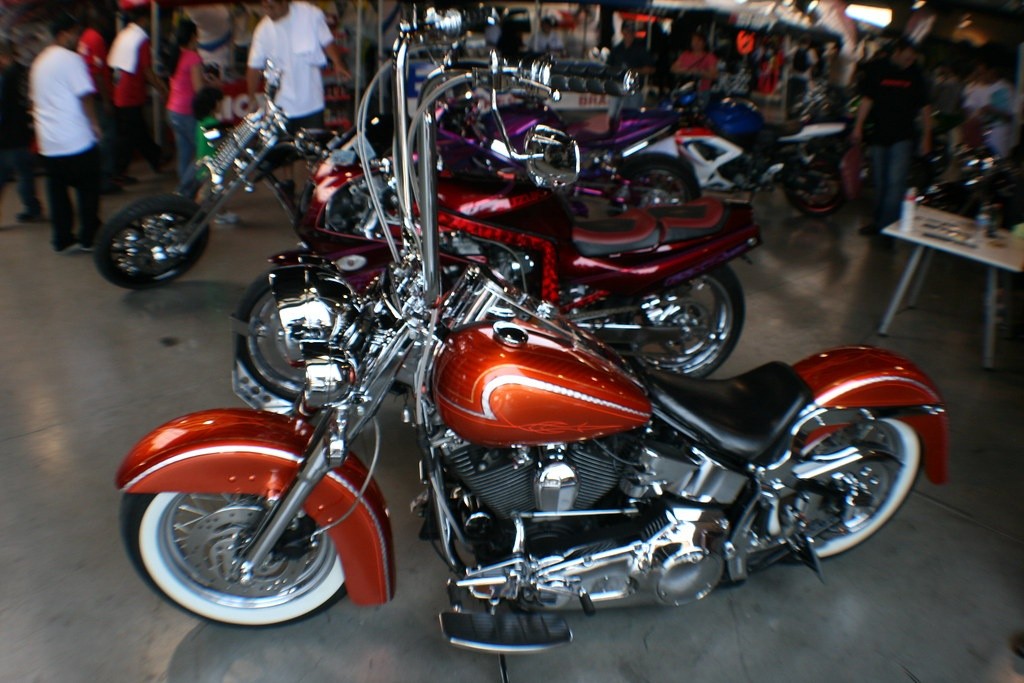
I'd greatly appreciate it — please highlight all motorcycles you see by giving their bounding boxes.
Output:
[88,0,1024,683]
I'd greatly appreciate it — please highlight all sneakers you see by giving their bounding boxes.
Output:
[214,209,240,224]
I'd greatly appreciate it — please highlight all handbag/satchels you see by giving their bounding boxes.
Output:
[841,143,864,199]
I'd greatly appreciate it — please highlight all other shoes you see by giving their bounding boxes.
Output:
[282,180,295,191]
[82,241,102,251]
[15,209,44,222]
[51,236,80,255]
[858,220,883,234]
[112,173,137,185]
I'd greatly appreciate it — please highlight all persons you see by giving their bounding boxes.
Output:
[0,0,352,255]
[470,2,817,131]
[852,29,1016,252]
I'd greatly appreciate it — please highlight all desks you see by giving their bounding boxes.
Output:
[876,206,1024,373]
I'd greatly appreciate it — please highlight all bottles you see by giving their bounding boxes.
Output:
[900,187,917,234]
[975,207,991,243]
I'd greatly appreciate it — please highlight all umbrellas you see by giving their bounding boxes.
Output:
[652,0,856,93]
